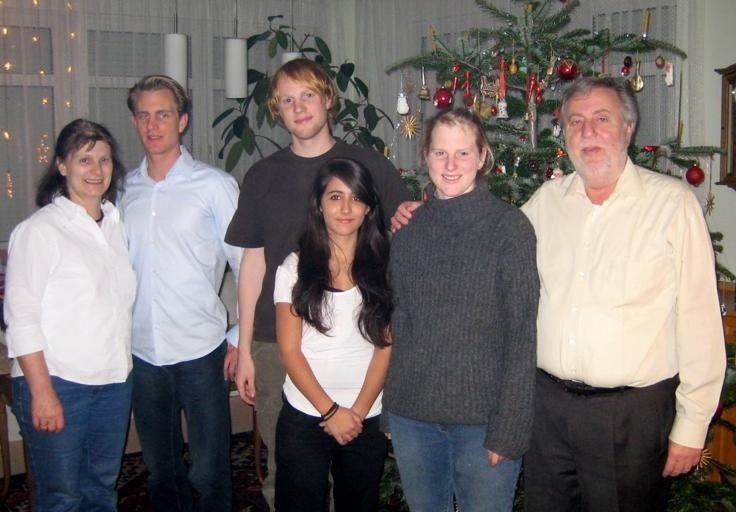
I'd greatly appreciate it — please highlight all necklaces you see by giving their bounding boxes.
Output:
[91,211,107,226]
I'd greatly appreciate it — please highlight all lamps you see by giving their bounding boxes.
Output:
[164,0,188,90]
[282,0,301,66]
[225,0,247,97]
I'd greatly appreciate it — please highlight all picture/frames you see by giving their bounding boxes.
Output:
[714,62,736,190]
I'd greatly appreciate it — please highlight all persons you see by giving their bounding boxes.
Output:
[272,158,392,510]
[223,59,422,509]
[109,76,247,510]
[3,118,137,510]
[391,76,727,510]
[378,105,538,510]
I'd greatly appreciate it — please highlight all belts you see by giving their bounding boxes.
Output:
[540,369,634,397]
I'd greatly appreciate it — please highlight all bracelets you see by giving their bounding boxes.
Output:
[320,403,337,422]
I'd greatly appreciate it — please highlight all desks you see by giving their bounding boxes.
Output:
[1,341,264,485]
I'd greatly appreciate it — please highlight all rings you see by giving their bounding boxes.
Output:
[40,423,48,426]
